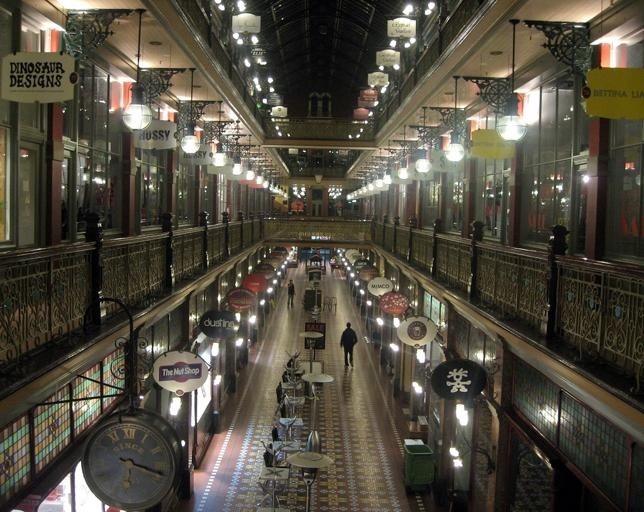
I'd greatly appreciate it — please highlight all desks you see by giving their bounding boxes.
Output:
[255,251,340,512]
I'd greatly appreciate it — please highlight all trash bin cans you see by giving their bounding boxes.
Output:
[404,438,435,485]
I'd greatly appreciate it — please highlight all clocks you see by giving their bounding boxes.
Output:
[76,408,186,511]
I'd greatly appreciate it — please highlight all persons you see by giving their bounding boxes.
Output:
[328,256,336,272]
[286,279,296,309]
[339,322,358,369]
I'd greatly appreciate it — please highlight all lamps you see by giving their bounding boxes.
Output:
[414,105,464,174]
[344,124,417,202]
[138,67,202,155]
[66,7,154,135]
[444,75,510,164]
[493,15,590,143]
[448,403,494,477]
[205,119,294,201]
[177,100,227,169]
